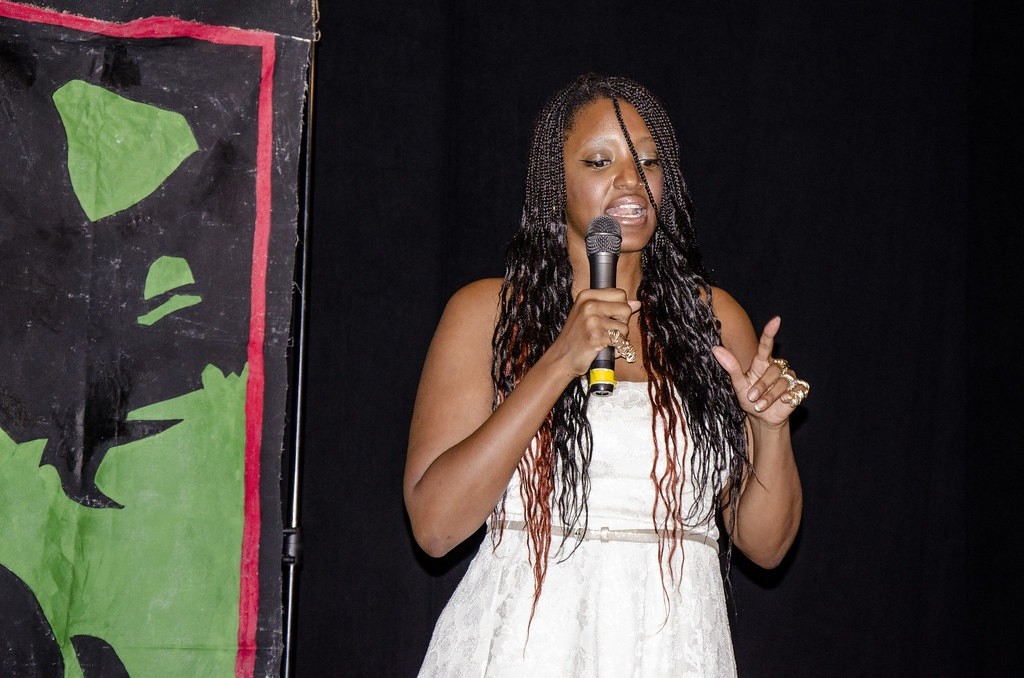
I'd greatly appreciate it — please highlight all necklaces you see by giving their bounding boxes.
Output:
[617,338,636,363]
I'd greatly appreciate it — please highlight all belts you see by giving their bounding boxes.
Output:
[484,519,720,555]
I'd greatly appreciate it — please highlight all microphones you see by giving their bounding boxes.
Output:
[583,215,623,399]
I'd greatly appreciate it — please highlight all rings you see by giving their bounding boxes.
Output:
[790,389,804,407]
[796,379,810,398]
[770,359,790,374]
[778,372,796,391]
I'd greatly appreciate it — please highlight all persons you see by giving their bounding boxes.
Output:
[401,72,811,678]
[608,329,620,346]
